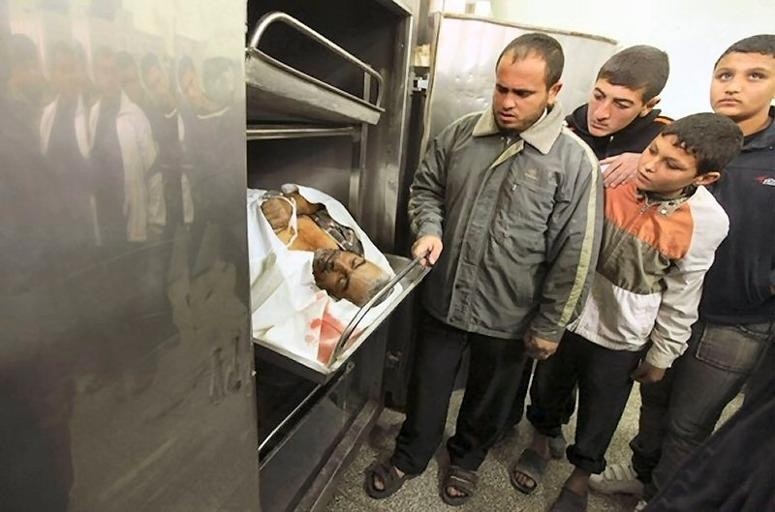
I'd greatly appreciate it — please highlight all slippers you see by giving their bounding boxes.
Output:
[550,487,587,512]
[512,448,552,494]
[366,458,419,498]
[441,466,478,506]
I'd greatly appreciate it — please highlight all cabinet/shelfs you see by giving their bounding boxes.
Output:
[0,2,617,512]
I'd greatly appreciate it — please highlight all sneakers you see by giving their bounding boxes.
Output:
[550,433,567,459]
[588,461,645,500]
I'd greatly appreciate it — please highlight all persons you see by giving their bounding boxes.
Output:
[509,111,744,512]
[587,32,774,512]
[261,189,393,310]
[364,33,605,506]
[490,45,676,460]
